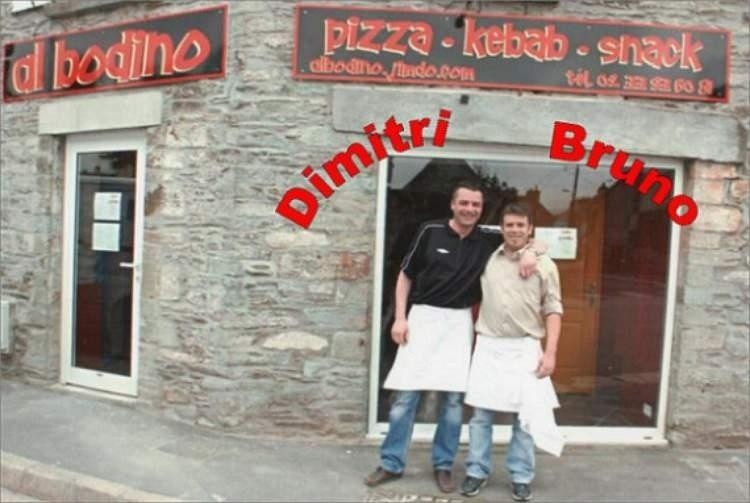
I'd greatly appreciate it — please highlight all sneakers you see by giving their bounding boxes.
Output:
[460,476,487,496]
[512,482,532,500]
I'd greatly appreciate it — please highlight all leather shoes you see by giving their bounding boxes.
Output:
[434,470,454,492]
[365,468,402,486]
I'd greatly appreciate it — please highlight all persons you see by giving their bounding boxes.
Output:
[363,175,550,494]
[459,200,565,501]
